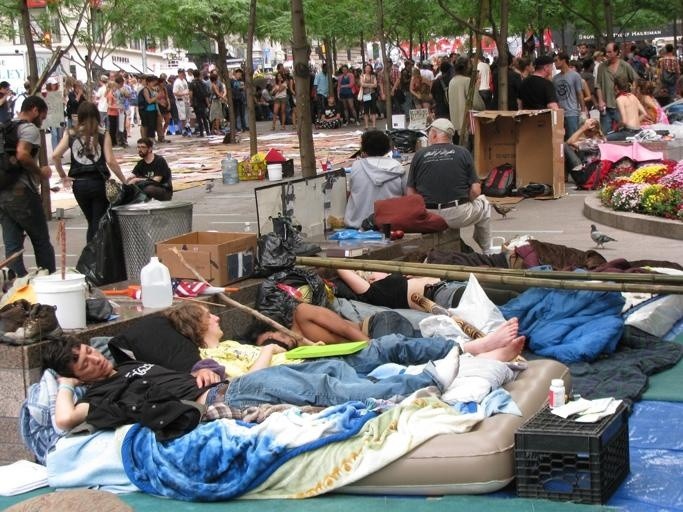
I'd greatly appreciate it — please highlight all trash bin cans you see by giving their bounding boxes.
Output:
[111,201,192,279]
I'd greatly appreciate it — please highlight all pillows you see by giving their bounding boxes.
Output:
[107,316,202,374]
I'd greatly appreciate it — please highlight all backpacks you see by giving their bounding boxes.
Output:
[0,119,39,191]
[575,160,612,190]
[513,181,554,199]
[482,161,516,196]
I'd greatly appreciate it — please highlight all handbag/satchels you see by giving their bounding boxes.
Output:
[104,179,121,203]
[363,94,372,102]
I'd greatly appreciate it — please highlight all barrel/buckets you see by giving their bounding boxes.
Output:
[140,257,172,308]
[32,274,87,328]
[267,164,283,181]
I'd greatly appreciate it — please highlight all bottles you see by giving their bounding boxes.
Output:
[549,379,565,410]
[325,161,331,171]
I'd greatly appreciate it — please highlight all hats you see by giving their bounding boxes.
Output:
[46,77,58,84]
[424,118,455,136]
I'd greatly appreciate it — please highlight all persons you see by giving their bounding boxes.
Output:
[402,250,523,271]
[254,58,415,130]
[410,38,683,183]
[125,137,174,202]
[1,68,246,158]
[339,128,409,230]
[0,94,55,280]
[162,298,528,379]
[332,268,531,314]
[50,99,128,245]
[404,115,502,255]
[37,335,461,435]
[240,302,423,351]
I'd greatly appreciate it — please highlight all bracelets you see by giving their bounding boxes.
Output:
[57,384,75,395]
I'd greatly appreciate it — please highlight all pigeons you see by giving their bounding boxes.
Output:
[206,177,216,192]
[491,202,517,219]
[589,223,616,249]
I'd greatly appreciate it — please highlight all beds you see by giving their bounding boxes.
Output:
[614,285,682,339]
[46,355,572,497]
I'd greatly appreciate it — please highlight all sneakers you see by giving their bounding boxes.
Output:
[0,302,64,345]
[482,246,501,257]
[0,298,32,343]
[111,113,382,150]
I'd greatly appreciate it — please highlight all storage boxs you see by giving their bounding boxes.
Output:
[153,230,258,288]
[472,108,565,205]
[267,159,294,180]
[238,155,266,181]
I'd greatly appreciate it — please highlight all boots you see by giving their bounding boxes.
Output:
[272,216,322,257]
[256,233,296,267]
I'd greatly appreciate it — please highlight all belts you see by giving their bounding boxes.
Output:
[213,382,229,402]
